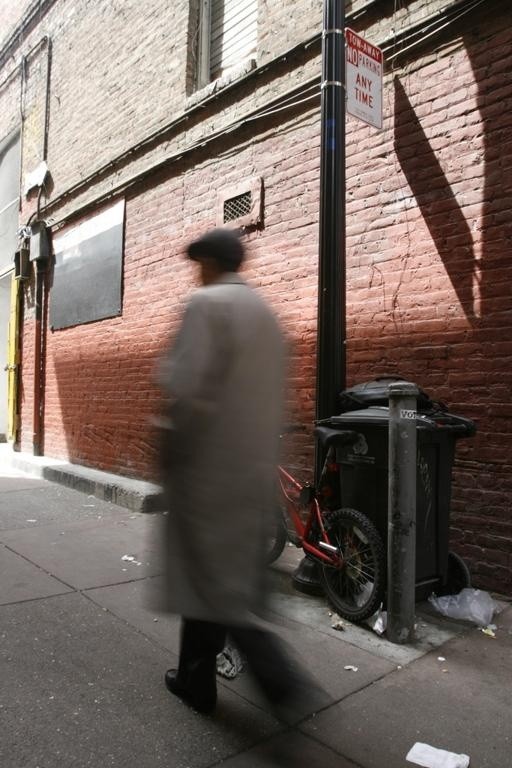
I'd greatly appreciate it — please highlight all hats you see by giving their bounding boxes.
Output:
[189,229,242,271]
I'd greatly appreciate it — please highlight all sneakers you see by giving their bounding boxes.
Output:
[165,668,215,716]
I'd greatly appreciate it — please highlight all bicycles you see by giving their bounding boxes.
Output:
[268,422,385,619]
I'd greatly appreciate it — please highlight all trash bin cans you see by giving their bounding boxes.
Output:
[316,377,470,604]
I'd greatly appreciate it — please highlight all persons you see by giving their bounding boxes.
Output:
[142,228,305,714]
